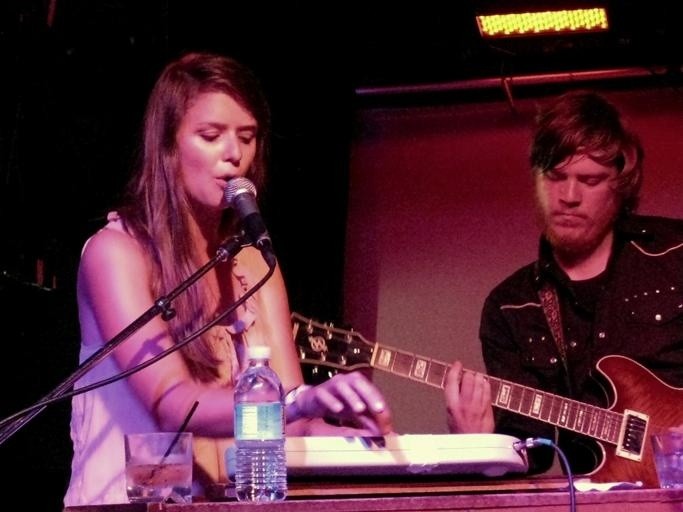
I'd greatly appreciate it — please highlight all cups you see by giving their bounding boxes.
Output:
[650,432,683,490]
[124,432,193,505]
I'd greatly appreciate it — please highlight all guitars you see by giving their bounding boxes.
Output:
[291,312,683,487]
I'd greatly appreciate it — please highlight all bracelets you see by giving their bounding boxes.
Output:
[283,382,312,413]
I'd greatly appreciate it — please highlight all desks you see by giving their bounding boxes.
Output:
[60,487,683,512]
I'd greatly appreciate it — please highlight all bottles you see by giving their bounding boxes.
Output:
[232,342,289,502]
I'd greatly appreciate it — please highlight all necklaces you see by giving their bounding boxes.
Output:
[206,255,259,339]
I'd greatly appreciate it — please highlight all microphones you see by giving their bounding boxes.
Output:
[225,173,279,272]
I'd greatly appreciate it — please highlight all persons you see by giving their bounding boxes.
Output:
[441,92,681,475]
[60,51,398,512]
